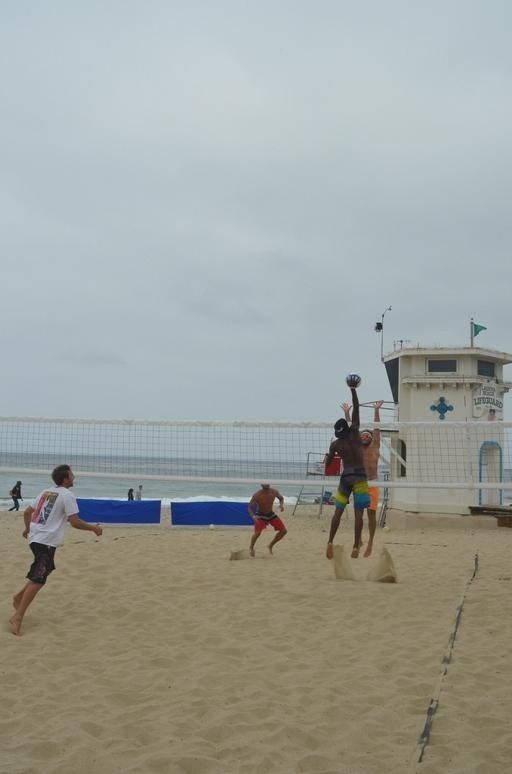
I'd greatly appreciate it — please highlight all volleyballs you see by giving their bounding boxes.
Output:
[346,374,361,388]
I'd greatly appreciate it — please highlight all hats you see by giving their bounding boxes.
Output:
[333,418,350,438]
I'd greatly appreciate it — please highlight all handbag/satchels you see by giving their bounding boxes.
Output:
[9,490,18,496]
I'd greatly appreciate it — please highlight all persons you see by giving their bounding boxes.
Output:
[324,374,371,558]
[339,399,384,557]
[8,480,24,511]
[247,483,288,557]
[136,484,144,501]
[128,487,134,500]
[8,464,103,636]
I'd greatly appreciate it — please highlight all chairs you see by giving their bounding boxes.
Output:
[292,452,348,518]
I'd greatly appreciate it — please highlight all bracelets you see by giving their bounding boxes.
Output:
[252,515,256,519]
[349,385,355,389]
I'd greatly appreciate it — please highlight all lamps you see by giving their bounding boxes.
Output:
[375,322,382,333]
